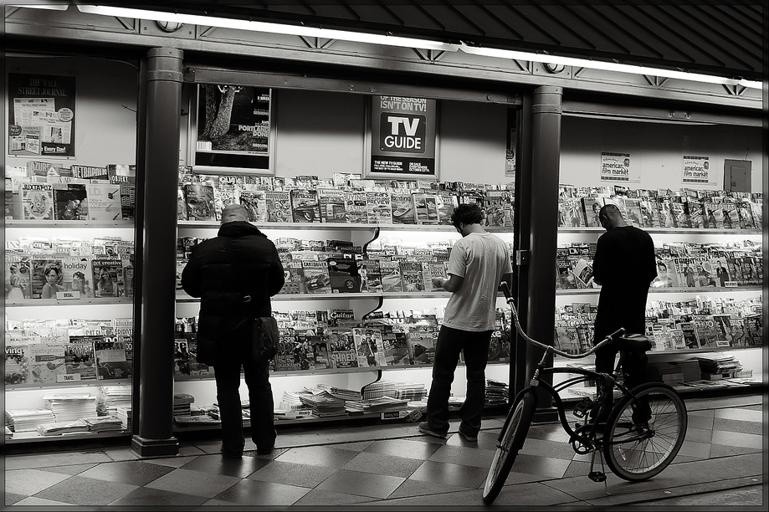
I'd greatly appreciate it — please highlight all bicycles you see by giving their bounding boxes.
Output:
[483,281,691,508]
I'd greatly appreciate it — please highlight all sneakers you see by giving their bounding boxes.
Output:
[575,420,608,433]
[459,427,477,441]
[419,422,447,438]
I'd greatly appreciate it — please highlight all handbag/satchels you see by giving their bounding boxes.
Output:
[251,317,280,361]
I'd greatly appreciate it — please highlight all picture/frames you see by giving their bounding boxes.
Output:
[4,69,79,161]
[361,97,443,182]
[186,83,280,178]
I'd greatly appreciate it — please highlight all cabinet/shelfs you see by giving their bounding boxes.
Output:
[174,169,519,435]
[6,166,139,448]
[553,188,763,406]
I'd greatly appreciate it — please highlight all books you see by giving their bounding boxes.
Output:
[4,156,765,387]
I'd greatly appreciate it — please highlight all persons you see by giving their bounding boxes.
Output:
[182,202,285,458]
[419,202,513,442]
[588,204,658,431]
